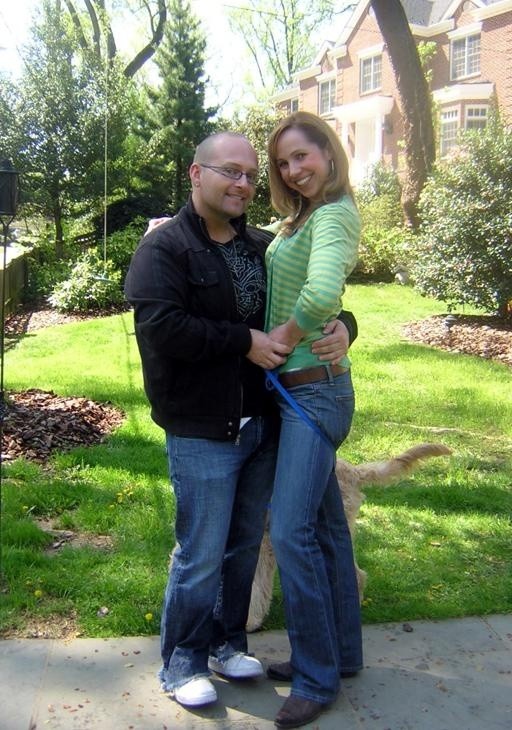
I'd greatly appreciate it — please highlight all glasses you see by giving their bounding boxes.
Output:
[198,163,256,185]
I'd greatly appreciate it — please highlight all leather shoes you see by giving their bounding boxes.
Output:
[273,694,324,727]
[266,661,359,683]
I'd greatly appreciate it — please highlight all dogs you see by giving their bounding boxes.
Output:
[167,441,454,632]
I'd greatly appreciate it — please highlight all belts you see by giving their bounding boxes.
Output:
[277,364,349,387]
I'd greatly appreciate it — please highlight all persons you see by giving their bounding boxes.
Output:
[122,133,359,709]
[257,110,364,729]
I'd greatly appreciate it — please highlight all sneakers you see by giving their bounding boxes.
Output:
[171,675,218,708]
[207,641,264,678]
